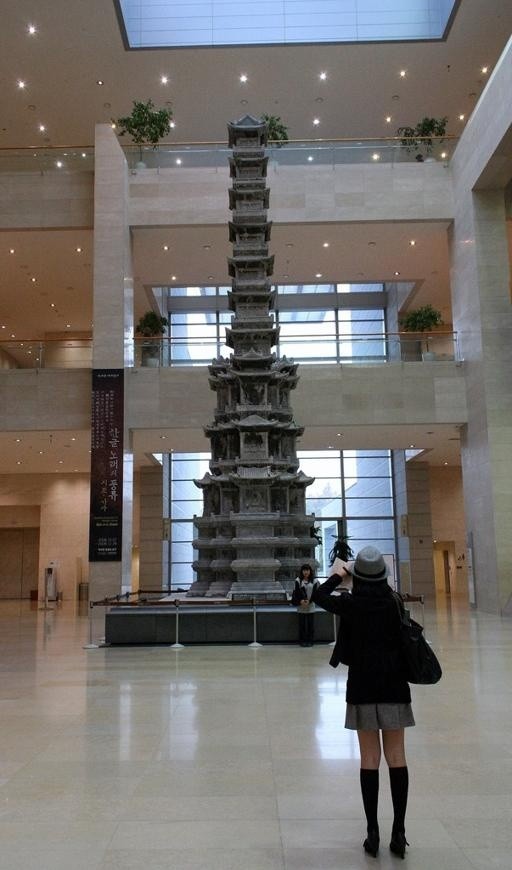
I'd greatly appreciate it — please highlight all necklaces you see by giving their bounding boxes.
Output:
[341,565,350,576]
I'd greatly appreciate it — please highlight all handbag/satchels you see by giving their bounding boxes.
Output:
[292,577,308,606]
[392,592,443,685]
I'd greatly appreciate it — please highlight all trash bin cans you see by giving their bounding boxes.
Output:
[44,567,57,601]
[80,583,89,600]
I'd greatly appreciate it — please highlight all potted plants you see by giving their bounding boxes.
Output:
[136,311,168,368]
[396,114,450,164]
[398,301,442,360]
[114,98,172,170]
[254,114,288,165]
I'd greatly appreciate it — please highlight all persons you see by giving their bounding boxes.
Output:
[295,563,320,648]
[309,544,415,858]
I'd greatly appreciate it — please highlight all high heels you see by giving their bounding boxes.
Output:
[389,834,408,859]
[363,837,380,858]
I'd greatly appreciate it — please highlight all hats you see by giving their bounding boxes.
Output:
[350,545,390,581]
